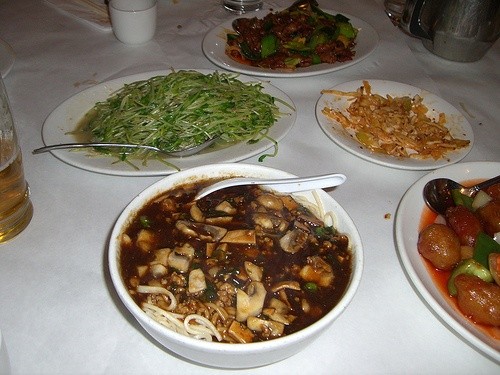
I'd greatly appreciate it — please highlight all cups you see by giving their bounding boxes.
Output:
[108,0,157,43]
[224,0,262,15]
[401,0,500,63]
[0,73,33,244]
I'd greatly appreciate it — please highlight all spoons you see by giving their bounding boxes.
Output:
[195,174,346,201]
[33,133,224,156]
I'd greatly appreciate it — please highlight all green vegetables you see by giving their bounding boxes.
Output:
[225,11,355,67]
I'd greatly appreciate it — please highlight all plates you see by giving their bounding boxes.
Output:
[316,80,474,170]
[396,162,500,360]
[203,8,379,78]
[42,69,296,176]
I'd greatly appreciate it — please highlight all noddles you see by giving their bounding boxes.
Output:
[119,177,354,343]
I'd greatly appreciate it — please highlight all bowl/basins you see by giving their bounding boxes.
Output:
[109,164,363,368]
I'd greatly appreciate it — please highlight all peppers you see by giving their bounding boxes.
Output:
[472,231,500,270]
[451,189,475,212]
[448,260,494,296]
